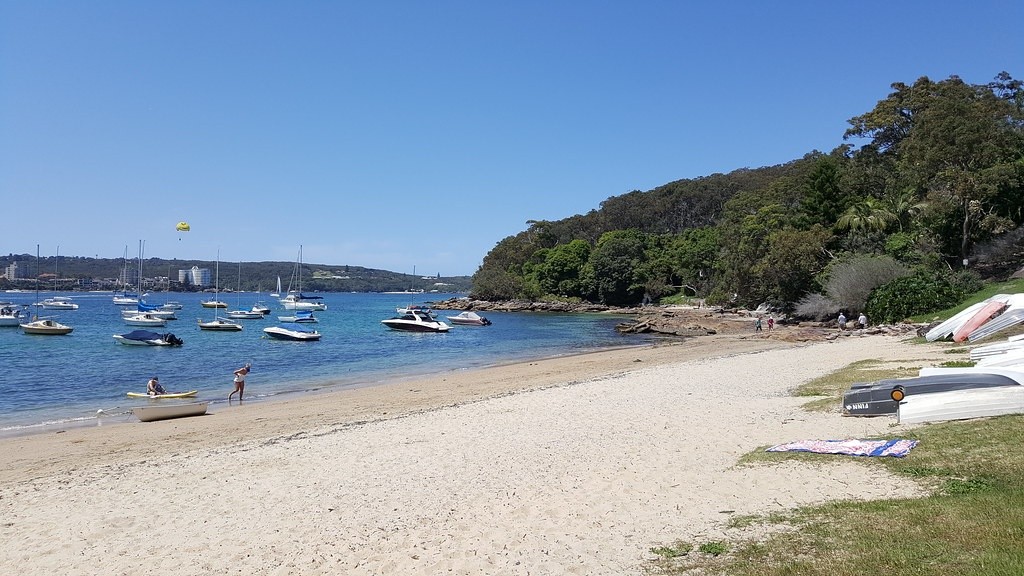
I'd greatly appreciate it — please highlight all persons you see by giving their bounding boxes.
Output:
[858,313,866,329]
[147,376,168,395]
[838,313,846,329]
[228,366,250,400]
[755,318,762,333]
[768,316,774,333]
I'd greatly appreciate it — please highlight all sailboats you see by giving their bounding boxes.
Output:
[20,244,73,334]
[33,245,79,309]
[199,250,245,331]
[395,265,438,317]
[113,240,183,326]
[226,261,264,319]
[271,245,328,311]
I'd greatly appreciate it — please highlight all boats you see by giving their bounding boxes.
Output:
[126,390,198,399]
[251,303,271,314]
[263,323,322,341]
[200,301,227,308]
[446,311,491,327]
[112,330,177,345]
[278,310,321,322]
[131,401,209,421]
[0,299,27,326]
[381,310,454,332]
[841,297,1024,426]
